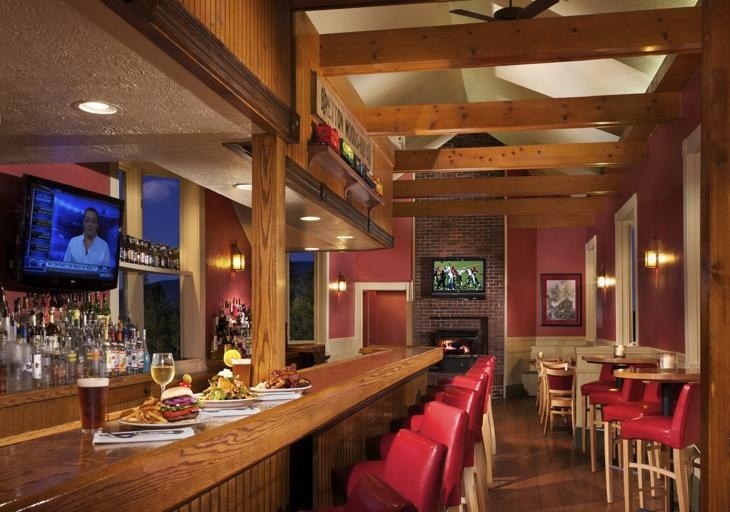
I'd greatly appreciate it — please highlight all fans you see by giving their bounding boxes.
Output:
[451,0,559,22]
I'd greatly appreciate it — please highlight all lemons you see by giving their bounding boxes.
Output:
[223,349,241,368]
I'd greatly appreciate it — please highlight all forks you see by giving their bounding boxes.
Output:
[105,429,184,438]
[203,406,249,413]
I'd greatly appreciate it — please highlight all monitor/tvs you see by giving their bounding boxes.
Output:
[13,172,126,291]
[431,257,486,298]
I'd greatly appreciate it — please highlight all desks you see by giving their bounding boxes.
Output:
[613,367,700,511]
[582,354,657,466]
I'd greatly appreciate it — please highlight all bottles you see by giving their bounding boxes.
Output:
[119,232,185,269]
[0,288,150,393]
[211,294,256,360]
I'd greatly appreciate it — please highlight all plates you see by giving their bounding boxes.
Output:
[251,384,313,398]
[118,409,212,427]
[190,392,264,407]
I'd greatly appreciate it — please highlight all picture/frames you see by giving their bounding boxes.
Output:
[540,272,582,327]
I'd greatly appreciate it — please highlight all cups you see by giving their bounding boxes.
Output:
[615,345,626,360]
[77,379,109,433]
[151,352,175,397]
[232,359,251,389]
[656,352,677,372]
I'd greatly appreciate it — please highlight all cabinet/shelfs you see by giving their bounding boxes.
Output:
[208,351,298,367]
[1,373,153,438]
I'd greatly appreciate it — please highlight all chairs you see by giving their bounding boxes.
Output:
[407,369,494,488]
[603,369,684,504]
[323,429,449,511]
[379,385,486,512]
[346,401,469,511]
[581,364,617,459]
[621,382,701,512]
[390,376,488,506]
[433,354,496,400]
[420,358,496,454]
[531,352,579,429]
[589,365,656,473]
[345,472,419,512]
[544,367,578,439]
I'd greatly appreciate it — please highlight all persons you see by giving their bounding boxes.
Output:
[471,265,482,287]
[63,208,111,267]
[435,267,446,290]
[451,264,463,286]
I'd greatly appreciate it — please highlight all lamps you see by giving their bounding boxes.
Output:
[641,238,658,268]
[232,243,246,271]
[338,273,346,292]
